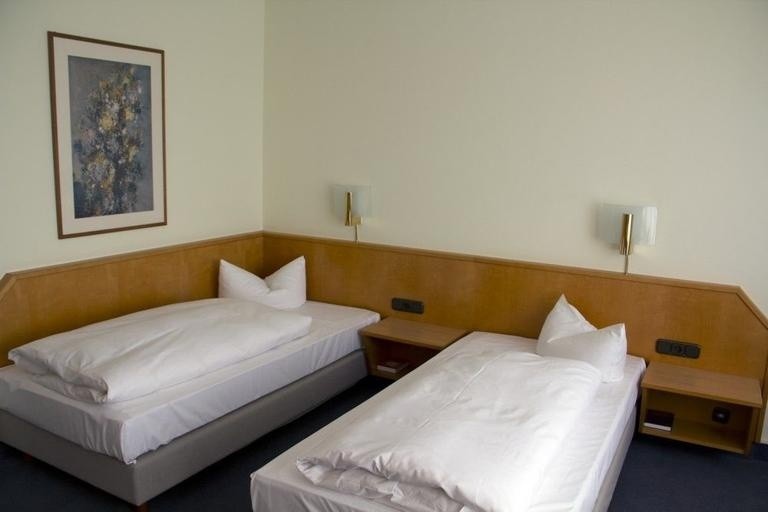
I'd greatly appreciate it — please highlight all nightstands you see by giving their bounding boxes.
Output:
[638,360,762,456]
[358,316,471,379]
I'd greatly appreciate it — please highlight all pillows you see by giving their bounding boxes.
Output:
[217,256,307,310]
[536,292,628,384]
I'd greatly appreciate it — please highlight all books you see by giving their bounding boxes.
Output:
[684,416,715,440]
[642,408,674,434]
[375,355,408,375]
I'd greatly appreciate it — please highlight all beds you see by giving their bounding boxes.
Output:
[0,299,381,512]
[250,332,646,511]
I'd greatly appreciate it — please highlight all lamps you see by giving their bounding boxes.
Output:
[329,184,381,240]
[599,204,657,275]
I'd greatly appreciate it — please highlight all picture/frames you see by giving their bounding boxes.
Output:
[47,30,167,240]
